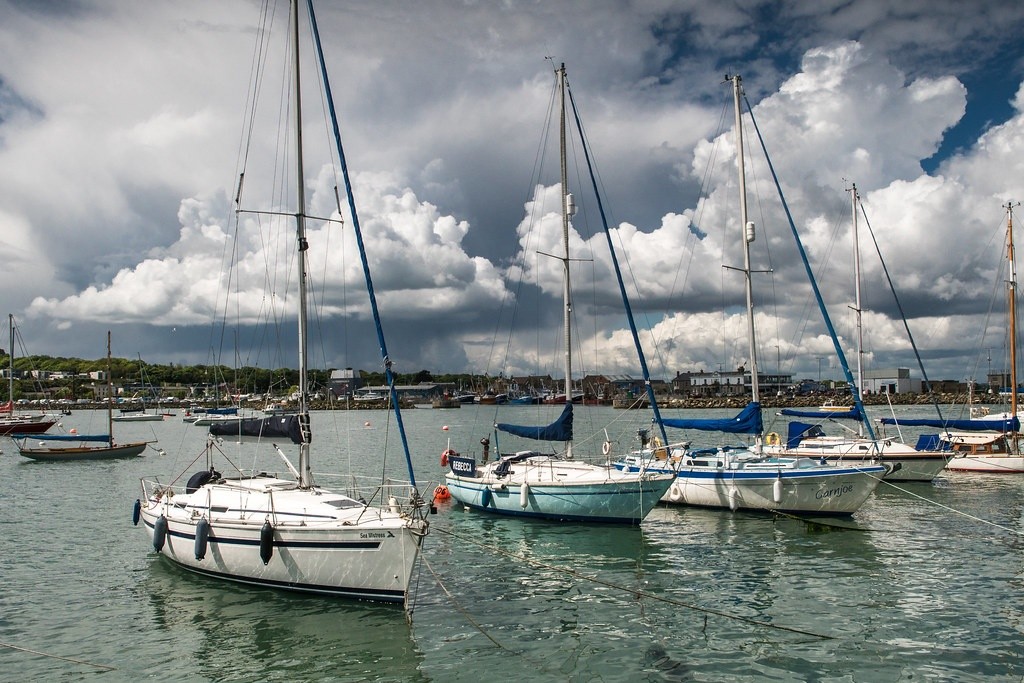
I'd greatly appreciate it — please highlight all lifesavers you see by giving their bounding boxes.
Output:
[602,441,610,455]
[767,431,780,445]
[440,449,455,468]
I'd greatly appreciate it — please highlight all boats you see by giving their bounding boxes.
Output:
[183,405,300,439]
[118,403,143,413]
[819,399,854,413]
[447,370,608,407]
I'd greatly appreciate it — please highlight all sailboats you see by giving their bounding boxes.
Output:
[610,73,897,516]
[133,3,434,609]
[0,313,62,434]
[761,180,961,483]
[440,58,686,527]
[924,201,1024,478]
[9,331,147,461]
[111,352,165,422]
